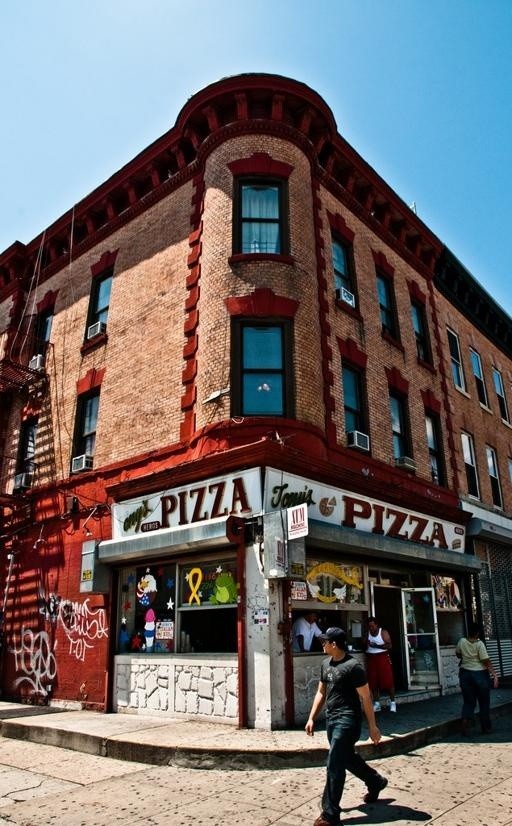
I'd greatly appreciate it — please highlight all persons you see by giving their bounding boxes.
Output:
[304,627,388,826]
[453,621,499,736]
[355,615,398,714]
[290,609,324,653]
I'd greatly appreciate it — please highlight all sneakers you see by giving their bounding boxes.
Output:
[313,813,340,825]
[463,720,493,736]
[389,701,396,712]
[363,776,388,803]
[373,701,381,711]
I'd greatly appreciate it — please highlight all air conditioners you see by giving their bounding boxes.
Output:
[396,454,418,472]
[71,454,94,475]
[87,320,107,340]
[14,472,32,490]
[27,354,46,374]
[337,287,357,310]
[346,430,371,453]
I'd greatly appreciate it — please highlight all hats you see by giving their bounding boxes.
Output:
[319,626,348,645]
